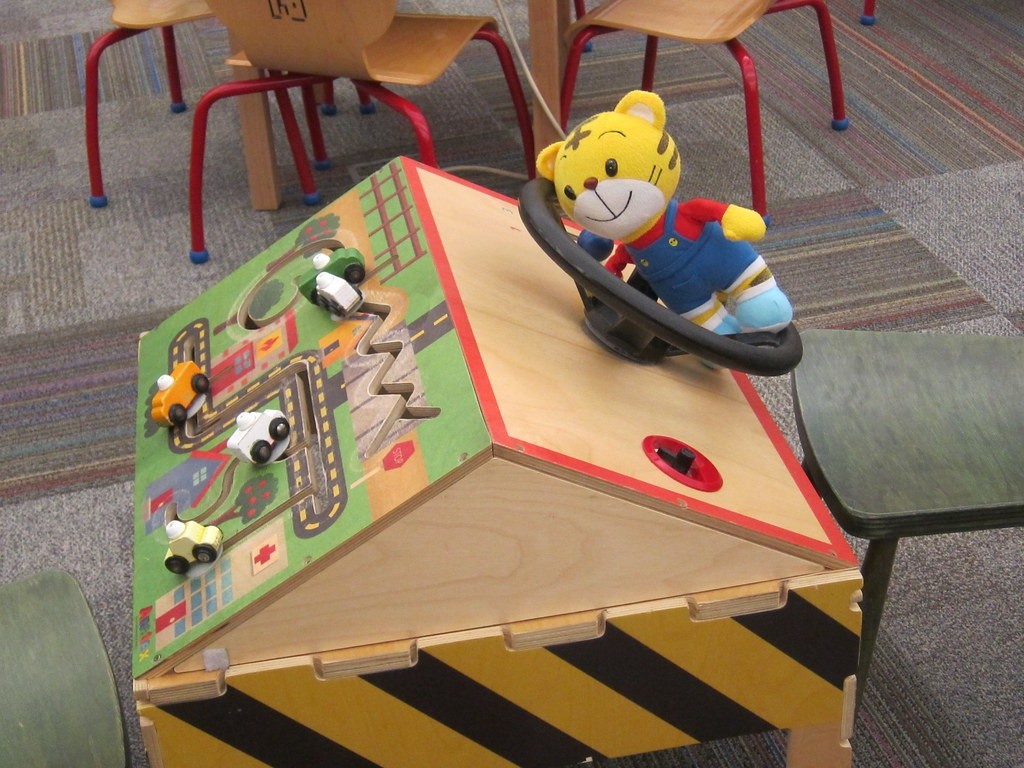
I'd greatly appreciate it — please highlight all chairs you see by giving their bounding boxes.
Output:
[188,0,536,263]
[559,0,847,228]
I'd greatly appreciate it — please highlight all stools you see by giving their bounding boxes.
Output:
[85,1,214,208]
[0,572,124,768]
[792,327,1023,721]
